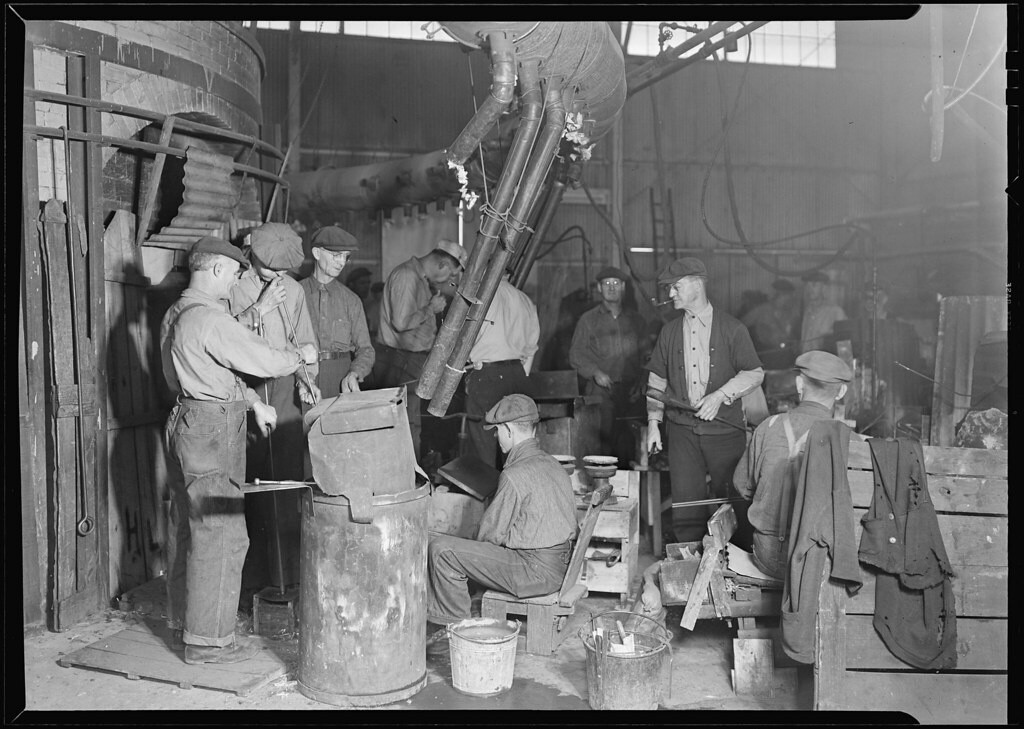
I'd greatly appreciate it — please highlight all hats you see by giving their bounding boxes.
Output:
[795,350,853,382]
[657,257,707,285]
[437,239,468,272]
[482,394,539,431]
[311,226,359,251]
[191,236,250,272]
[773,280,796,290]
[802,269,828,281]
[250,222,305,270]
[596,266,626,282]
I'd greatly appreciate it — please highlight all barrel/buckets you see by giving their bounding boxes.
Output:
[297,476,433,707]
[445,618,522,699]
[577,610,674,710]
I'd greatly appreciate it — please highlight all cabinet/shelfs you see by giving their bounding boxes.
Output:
[565,469,640,606]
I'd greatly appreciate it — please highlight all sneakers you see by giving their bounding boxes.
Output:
[184,643,258,665]
[426,628,451,655]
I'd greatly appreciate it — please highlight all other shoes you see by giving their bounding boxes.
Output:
[667,605,687,639]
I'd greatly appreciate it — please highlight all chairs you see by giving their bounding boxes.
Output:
[481,484,613,655]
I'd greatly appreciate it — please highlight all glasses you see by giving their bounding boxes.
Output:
[602,281,622,287]
[318,248,352,263]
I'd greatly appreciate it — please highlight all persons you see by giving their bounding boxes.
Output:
[425,394,580,655]
[732,350,852,581]
[645,256,765,554]
[740,279,800,370]
[799,273,849,356]
[225,222,322,603]
[464,259,540,468]
[371,240,468,463]
[569,266,655,471]
[296,226,375,399]
[346,267,373,300]
[159,234,318,664]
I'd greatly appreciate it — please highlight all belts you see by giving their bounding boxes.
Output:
[318,350,350,362]
